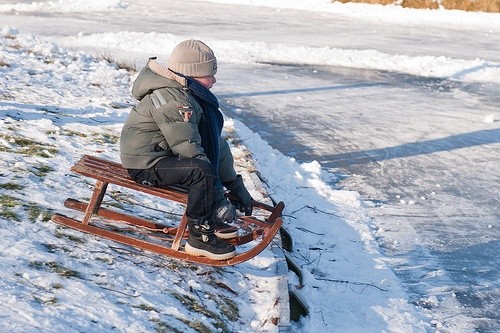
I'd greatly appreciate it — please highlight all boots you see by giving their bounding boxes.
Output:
[214,215,238,239]
[184,211,236,260]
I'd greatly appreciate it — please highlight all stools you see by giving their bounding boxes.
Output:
[70,154,190,251]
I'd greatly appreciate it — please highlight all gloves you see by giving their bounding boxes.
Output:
[213,186,237,223]
[224,175,254,216]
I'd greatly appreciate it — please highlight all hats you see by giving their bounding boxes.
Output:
[168,40,217,77]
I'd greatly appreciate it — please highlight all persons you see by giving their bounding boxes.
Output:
[120,39,256,260]
